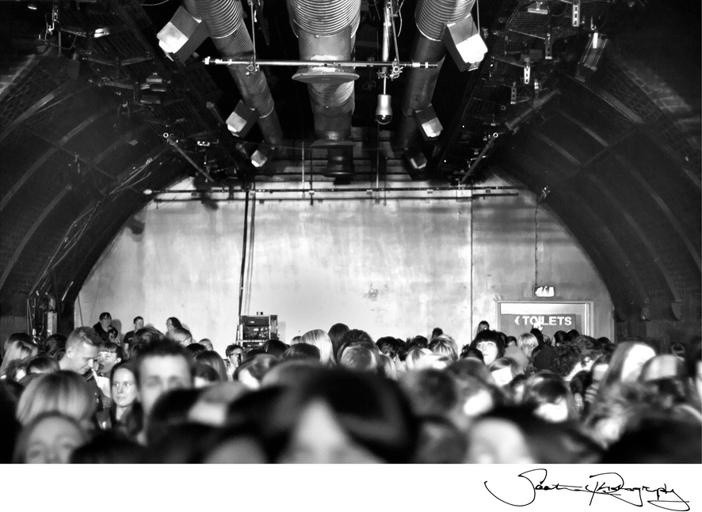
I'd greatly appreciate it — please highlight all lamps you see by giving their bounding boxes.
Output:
[250,140,276,170]
[225,98,256,138]
[158,7,201,56]
[413,104,444,141]
[375,0,394,127]
[446,13,487,77]
[408,151,428,171]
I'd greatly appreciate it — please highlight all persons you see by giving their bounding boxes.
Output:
[0,311,702,464]
